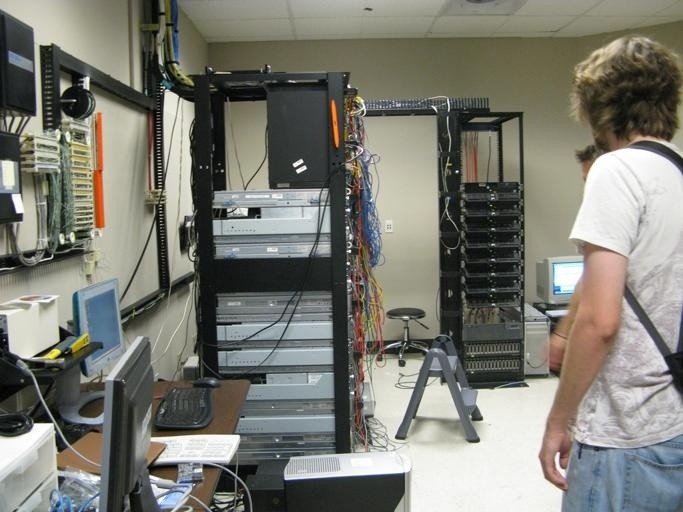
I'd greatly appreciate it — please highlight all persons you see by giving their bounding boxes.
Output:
[539,34,681,512]
[541,139,607,375]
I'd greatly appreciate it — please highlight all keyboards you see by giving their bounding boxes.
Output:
[546,310,569,317]
[153,387,215,430]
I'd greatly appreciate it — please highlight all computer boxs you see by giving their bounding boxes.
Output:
[266,83,329,188]
[524,303,551,377]
[282,451,412,512]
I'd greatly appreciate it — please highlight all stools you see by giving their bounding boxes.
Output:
[377,308,431,367]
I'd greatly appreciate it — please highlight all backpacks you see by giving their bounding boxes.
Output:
[623,141,683,394]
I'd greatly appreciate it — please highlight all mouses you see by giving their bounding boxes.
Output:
[192,377,222,388]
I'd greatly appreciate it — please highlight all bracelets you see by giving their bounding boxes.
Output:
[551,330,568,340]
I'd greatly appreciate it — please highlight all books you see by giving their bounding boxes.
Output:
[56,432,168,478]
[150,434,241,467]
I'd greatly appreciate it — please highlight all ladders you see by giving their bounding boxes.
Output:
[395,335,483,443]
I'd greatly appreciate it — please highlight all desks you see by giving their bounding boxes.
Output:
[29,379,250,512]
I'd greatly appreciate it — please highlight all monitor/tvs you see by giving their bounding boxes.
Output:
[72,278,126,378]
[99,336,160,512]
[536,256,584,304]
